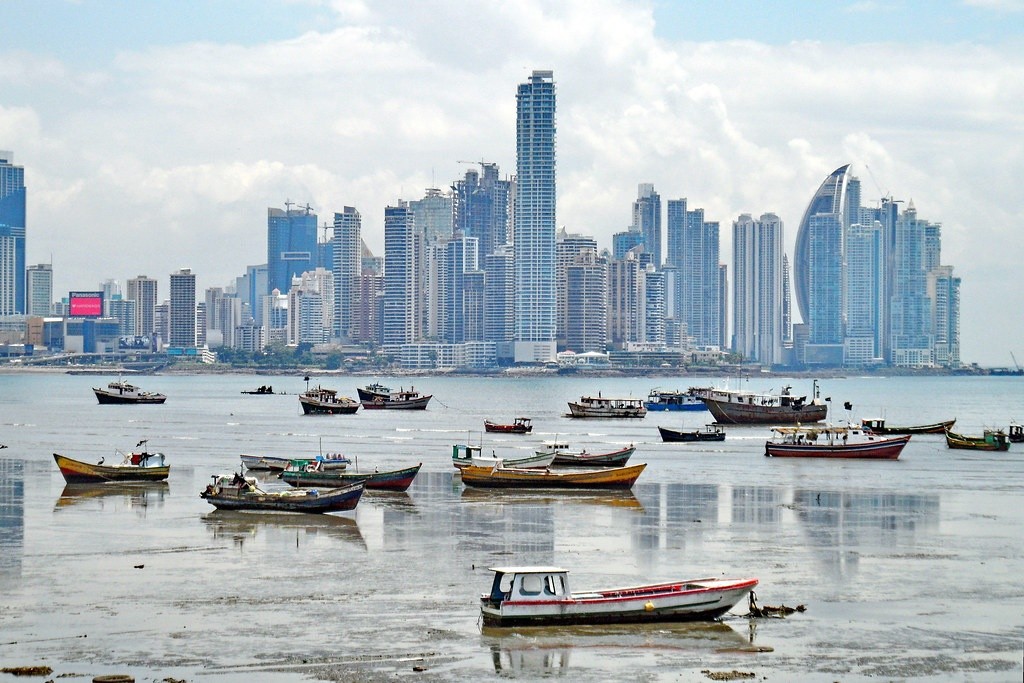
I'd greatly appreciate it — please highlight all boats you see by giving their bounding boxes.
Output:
[461,484,645,514]
[239,436,424,494]
[460,457,648,491]
[93,382,168,405]
[567,391,647,418]
[197,473,367,515]
[642,387,709,412]
[657,425,727,442]
[359,385,433,411]
[1008,423,1024,443]
[299,374,362,414]
[52,440,171,483]
[764,427,912,459]
[478,565,759,626]
[199,507,369,552]
[108,382,140,391]
[943,424,1011,452]
[535,433,636,468]
[861,418,957,436]
[356,380,392,402]
[240,385,277,395]
[483,416,533,433]
[51,477,171,514]
[477,619,775,680]
[694,378,827,424]
[451,443,558,472]
